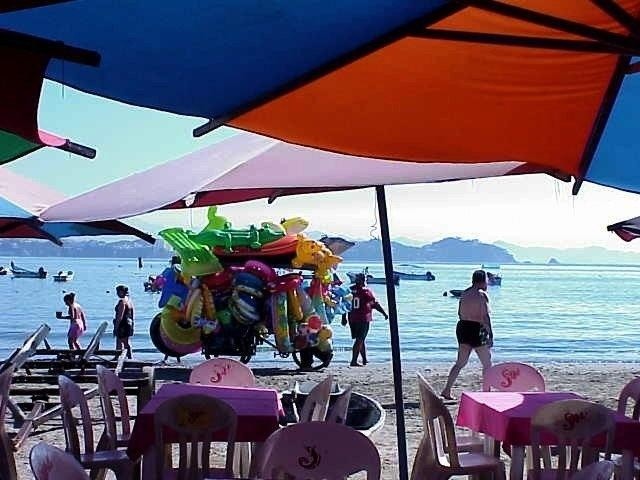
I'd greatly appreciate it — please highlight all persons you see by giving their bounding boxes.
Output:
[439,269,493,400]
[113,285,135,360]
[58,291,87,359]
[340,272,388,367]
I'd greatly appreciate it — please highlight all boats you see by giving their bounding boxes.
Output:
[472,263,501,269]
[346,271,386,284]
[0,260,74,282]
[279,381,387,439]
[393,271,436,282]
[487,271,503,288]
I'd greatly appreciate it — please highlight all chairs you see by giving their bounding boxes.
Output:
[329,383,354,424]
[0,362,17,479]
[618,376,640,421]
[279,374,333,428]
[438,414,484,452]
[410,373,506,480]
[28,443,90,480]
[526,399,616,479]
[57,375,140,479]
[482,364,546,452]
[189,358,256,388]
[248,421,380,480]
[155,393,239,479]
[91,365,132,478]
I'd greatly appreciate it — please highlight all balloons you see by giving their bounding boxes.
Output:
[151,203,353,353]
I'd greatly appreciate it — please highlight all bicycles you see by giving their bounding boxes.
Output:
[201,320,333,370]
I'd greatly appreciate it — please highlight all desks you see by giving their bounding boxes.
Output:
[455,390,640,480]
[127,384,278,480]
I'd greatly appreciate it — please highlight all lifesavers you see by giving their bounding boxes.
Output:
[193,204,354,274]
[150,227,222,357]
[203,259,352,354]
